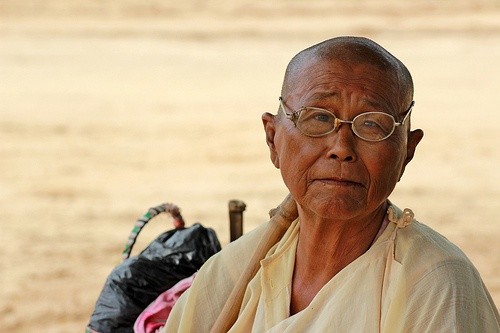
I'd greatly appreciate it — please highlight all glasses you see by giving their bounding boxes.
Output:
[279,94,416,142]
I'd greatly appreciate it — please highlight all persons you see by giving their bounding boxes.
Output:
[160,37,500,332]
